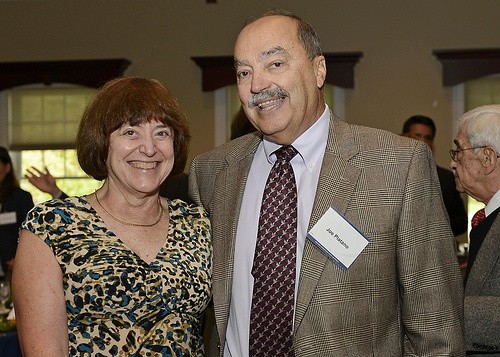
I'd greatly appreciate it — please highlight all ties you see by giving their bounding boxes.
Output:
[471,208,485,230]
[249,146,299,357]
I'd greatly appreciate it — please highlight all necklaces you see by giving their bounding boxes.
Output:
[95,189,163,227]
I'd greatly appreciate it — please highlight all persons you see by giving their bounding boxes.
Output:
[0,105,257,275]
[400,115,468,238]
[450,104,500,357]
[11,77,213,357]
[190,11,464,357]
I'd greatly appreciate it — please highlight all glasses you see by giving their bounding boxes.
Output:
[449,145,500,162]
